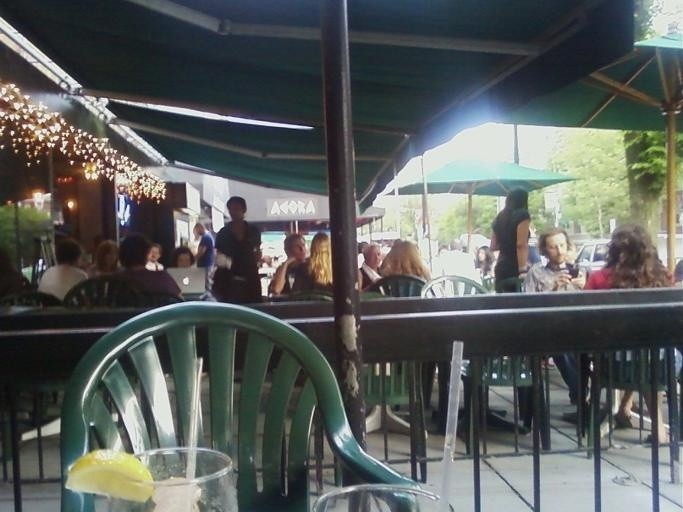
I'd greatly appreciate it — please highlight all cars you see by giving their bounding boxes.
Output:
[572,239,611,272]
[450,234,491,252]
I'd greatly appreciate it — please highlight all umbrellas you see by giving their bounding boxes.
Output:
[385,157,581,252]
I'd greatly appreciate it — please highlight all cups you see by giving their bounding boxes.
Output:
[93,447,239,511]
[311,484,454,512]
[570,277,584,291]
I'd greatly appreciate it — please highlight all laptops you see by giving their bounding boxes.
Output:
[166,267,206,295]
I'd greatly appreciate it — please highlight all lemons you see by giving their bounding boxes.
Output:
[64,449,154,503]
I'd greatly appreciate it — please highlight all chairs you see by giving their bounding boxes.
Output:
[0,270,681,512]
